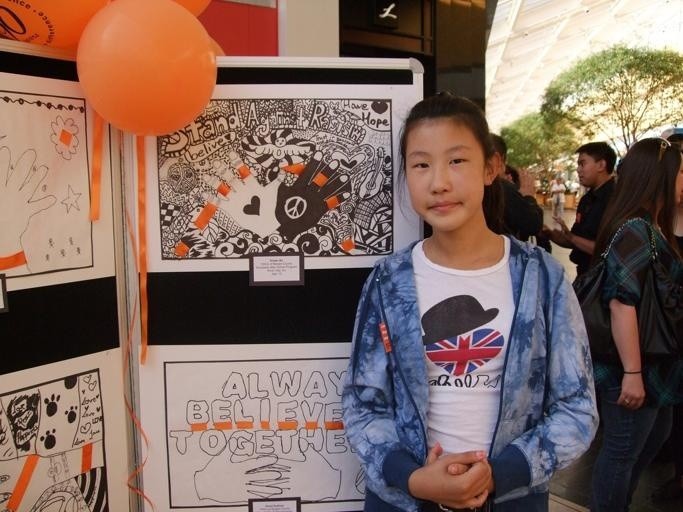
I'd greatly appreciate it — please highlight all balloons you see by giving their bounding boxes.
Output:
[74,1,218,144]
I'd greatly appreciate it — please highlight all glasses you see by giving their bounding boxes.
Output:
[655,137,671,162]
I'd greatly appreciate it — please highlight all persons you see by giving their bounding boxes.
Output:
[482,127,682,512]
[339,89,600,512]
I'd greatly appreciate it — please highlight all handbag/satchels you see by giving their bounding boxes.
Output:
[572,218,682,358]
